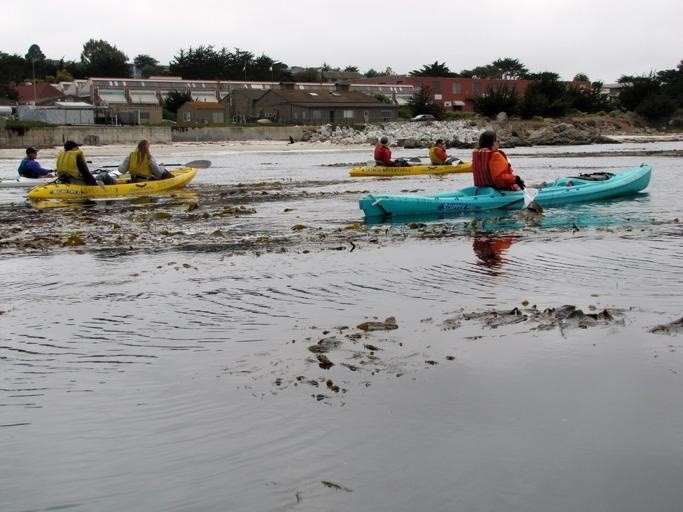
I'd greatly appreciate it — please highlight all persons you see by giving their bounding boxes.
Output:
[374,137,409,167]
[118,140,174,183]
[472,131,573,191]
[55,141,117,187]
[428,139,466,165]
[17,148,54,178]
[474,236,513,276]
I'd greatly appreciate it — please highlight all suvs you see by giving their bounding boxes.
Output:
[409,114,435,121]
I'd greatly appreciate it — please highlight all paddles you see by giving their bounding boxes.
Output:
[523,186,539,209]
[452,160,460,165]
[102,160,211,168]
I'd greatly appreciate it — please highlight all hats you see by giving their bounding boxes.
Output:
[380,136,389,144]
[64,140,83,149]
[25,147,40,153]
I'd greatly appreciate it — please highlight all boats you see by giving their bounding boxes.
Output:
[356,162,653,222]
[346,161,473,176]
[0,165,196,202]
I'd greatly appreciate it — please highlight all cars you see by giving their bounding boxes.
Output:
[161,119,175,125]
